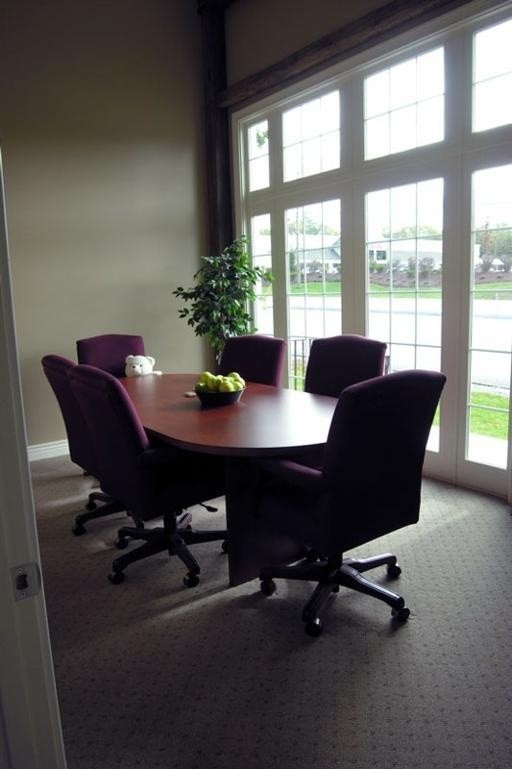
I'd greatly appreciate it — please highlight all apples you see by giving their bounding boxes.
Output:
[196,370,245,392]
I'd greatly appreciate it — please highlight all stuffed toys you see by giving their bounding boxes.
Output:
[123,354,162,378]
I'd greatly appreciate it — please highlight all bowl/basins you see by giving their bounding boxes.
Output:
[192,385,247,407]
